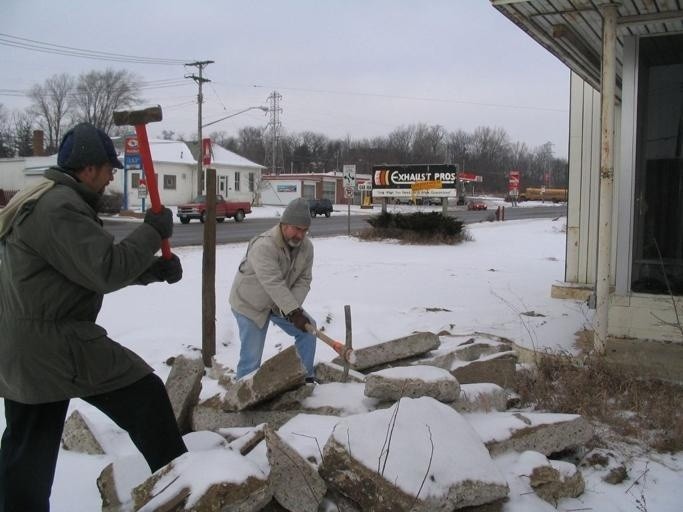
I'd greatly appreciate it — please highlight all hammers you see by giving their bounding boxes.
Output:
[112,105,173,261]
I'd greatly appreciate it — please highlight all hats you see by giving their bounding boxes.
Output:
[280,197,312,226]
[58,123,124,169]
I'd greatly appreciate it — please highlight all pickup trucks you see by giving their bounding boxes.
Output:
[177,193,251,224]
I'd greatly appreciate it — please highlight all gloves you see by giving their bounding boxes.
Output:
[144,205,173,239]
[294,315,310,332]
[161,254,182,283]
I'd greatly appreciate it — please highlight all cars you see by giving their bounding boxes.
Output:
[470,200,487,210]
[394,196,442,205]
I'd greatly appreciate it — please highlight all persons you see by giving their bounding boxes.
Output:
[227,197,324,387]
[0,122,190,511]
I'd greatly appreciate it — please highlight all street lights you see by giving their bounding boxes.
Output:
[198,105,268,196]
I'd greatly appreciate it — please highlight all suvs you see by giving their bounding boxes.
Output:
[309,199,333,217]
